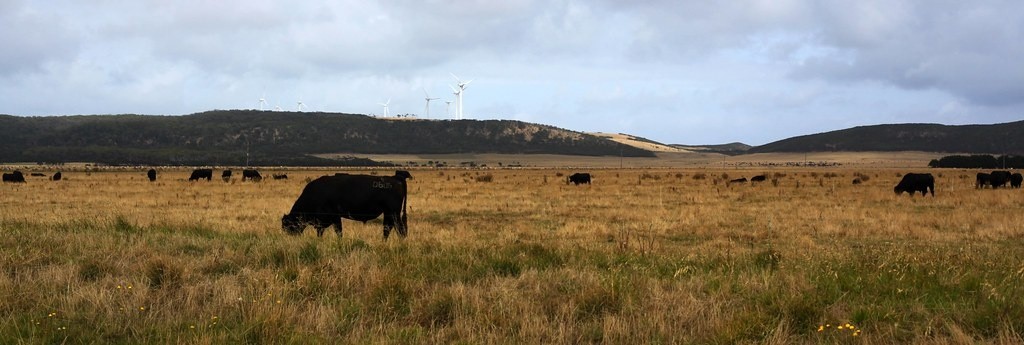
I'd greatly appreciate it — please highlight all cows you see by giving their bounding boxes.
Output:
[2,170,25,182]
[567,172,591,187]
[53,171,61,181]
[241,169,262,183]
[221,169,233,183]
[147,168,156,181]
[188,168,213,183]
[893,172,936,203]
[975,170,1024,190]
[281,172,408,240]
[730,175,766,183]
[395,169,413,180]
